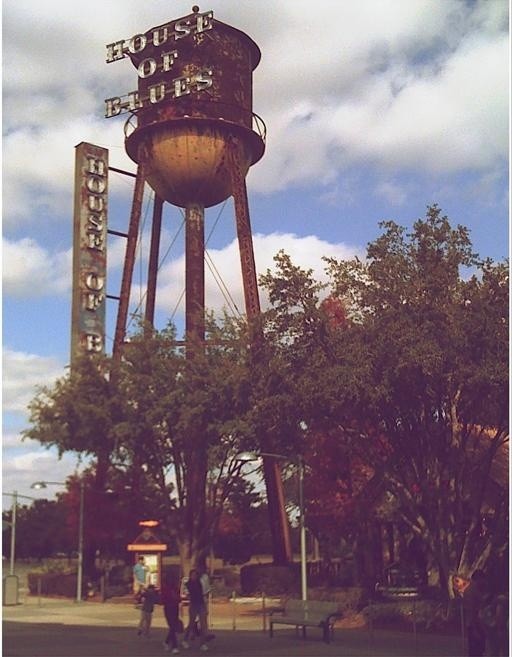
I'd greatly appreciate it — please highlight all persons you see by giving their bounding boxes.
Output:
[183,568,211,651]
[135,582,159,638]
[133,553,148,601]
[162,576,184,654]
[485,581,508,656]
[188,563,216,641]
[466,606,486,656]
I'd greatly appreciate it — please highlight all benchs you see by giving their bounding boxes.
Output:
[267,598,346,644]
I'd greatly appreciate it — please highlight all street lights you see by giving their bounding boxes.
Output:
[31,479,86,601]
[236,450,308,623]
